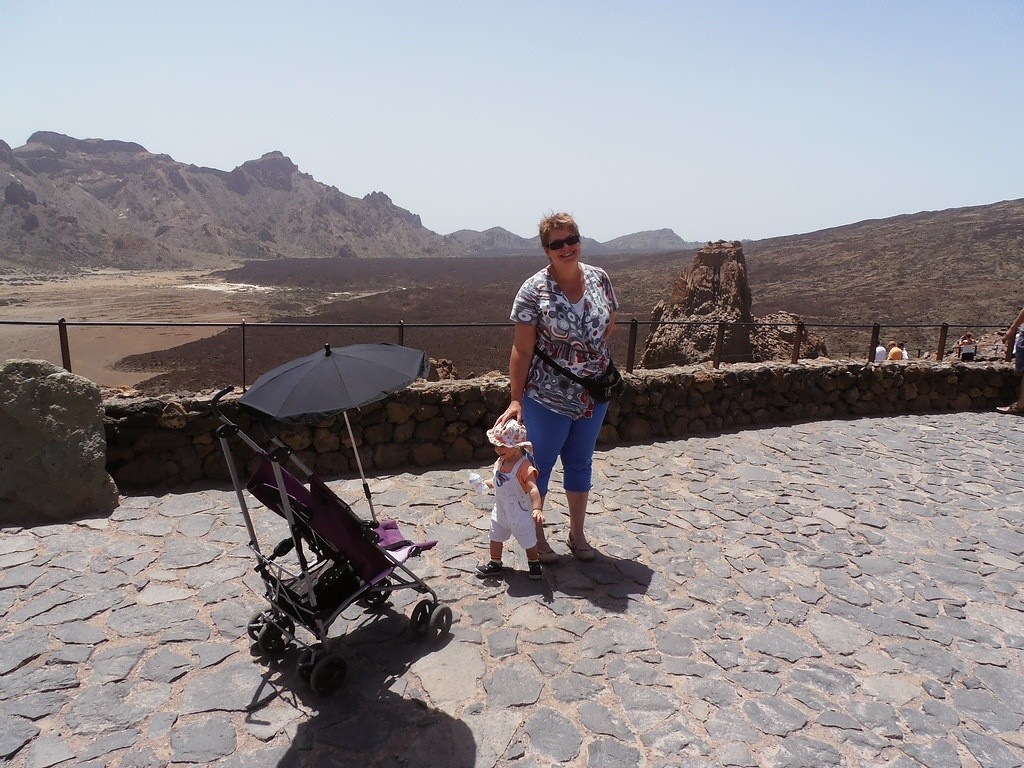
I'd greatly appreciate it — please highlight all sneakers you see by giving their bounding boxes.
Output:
[528,561,543,580]
[475,560,503,576]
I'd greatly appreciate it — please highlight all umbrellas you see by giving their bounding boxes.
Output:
[237,342,431,527]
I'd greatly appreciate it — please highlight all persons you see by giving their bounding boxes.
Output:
[493,212,619,564]
[995,308,1024,416]
[887,341,902,360]
[475,418,545,579]
[875,341,887,360]
[959,332,976,361]
[897,342,909,359]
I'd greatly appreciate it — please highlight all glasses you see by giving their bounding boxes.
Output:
[546,236,580,251]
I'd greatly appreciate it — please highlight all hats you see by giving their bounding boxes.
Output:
[486,418,534,455]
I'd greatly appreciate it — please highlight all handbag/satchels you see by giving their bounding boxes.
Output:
[584,361,625,404]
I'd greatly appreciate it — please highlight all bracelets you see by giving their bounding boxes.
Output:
[532,508,542,511]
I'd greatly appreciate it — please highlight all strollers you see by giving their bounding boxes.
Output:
[212,383,455,700]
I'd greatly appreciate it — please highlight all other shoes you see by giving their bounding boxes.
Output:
[996,402,1024,415]
[566,531,595,561]
[536,543,559,562]
